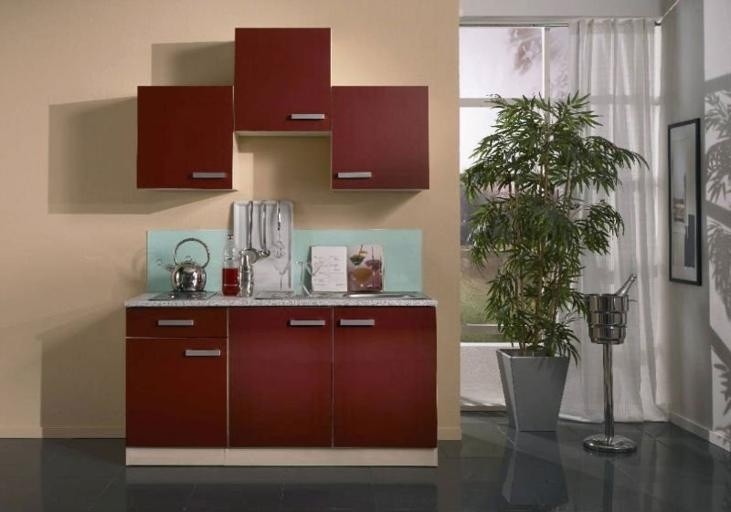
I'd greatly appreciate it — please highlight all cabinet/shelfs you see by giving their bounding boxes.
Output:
[331,86,429,193]
[135,85,236,191]
[234,28,331,137]
[123,307,229,467]
[230,305,334,468]
[335,305,438,469]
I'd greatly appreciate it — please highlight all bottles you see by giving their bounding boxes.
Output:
[614,272,636,297]
[222,235,254,297]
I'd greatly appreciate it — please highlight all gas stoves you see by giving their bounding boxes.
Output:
[147,290,217,300]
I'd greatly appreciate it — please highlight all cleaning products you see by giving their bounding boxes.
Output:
[223,233,240,294]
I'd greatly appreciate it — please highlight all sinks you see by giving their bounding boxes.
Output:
[254,296,341,301]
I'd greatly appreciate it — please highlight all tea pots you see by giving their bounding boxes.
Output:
[171,238,210,292]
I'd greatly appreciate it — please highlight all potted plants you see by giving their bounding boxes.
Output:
[459,89,650,433]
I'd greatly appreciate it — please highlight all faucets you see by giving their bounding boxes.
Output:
[237,245,270,298]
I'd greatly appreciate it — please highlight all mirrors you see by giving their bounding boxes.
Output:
[668,117,702,286]
